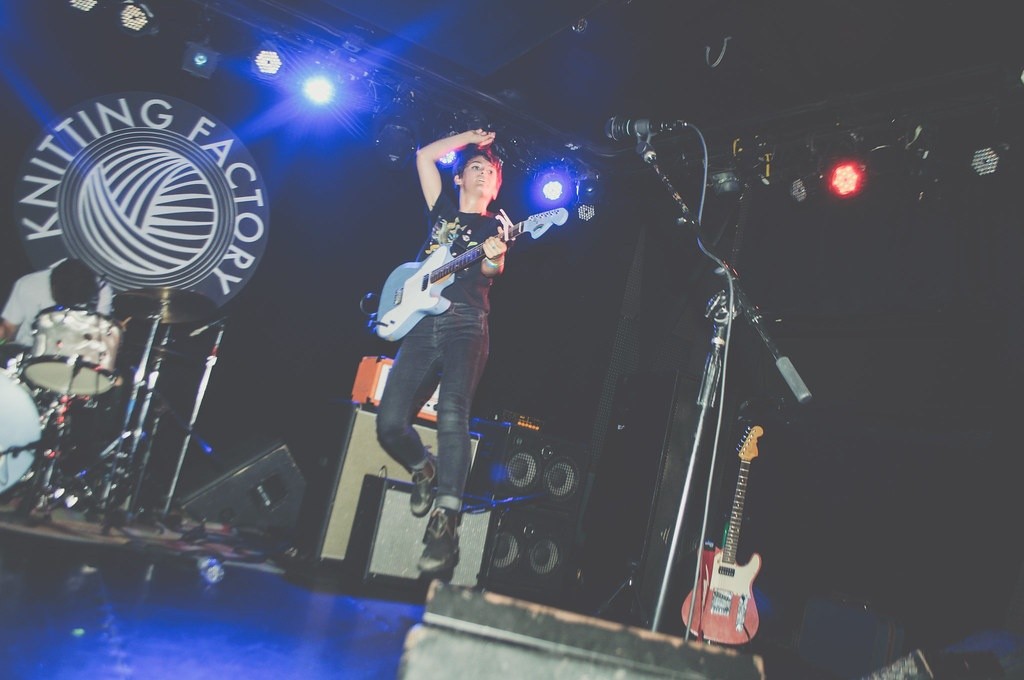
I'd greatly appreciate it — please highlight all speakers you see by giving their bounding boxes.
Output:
[178,441,305,546]
[317,406,764,680]
[859,648,1009,680]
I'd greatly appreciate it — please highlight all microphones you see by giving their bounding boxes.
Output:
[605,114,688,140]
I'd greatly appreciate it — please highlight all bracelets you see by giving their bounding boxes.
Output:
[484,257,504,269]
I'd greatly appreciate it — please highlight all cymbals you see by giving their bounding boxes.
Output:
[113,289,218,323]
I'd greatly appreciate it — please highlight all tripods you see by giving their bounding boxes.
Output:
[12,313,229,540]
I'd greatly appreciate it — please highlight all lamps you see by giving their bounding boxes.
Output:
[971,148,999,176]
[68,0,100,16]
[825,158,866,199]
[577,204,595,222]
[114,0,155,37]
[250,40,287,82]
[790,177,808,204]
[537,172,567,206]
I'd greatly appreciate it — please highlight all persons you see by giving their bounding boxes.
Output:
[375,128,515,575]
[0,255,113,348]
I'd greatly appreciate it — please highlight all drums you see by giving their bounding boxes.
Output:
[19,300,124,398]
[0,364,40,494]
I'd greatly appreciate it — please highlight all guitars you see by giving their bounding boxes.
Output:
[376,207,571,344]
[680,423,764,646]
